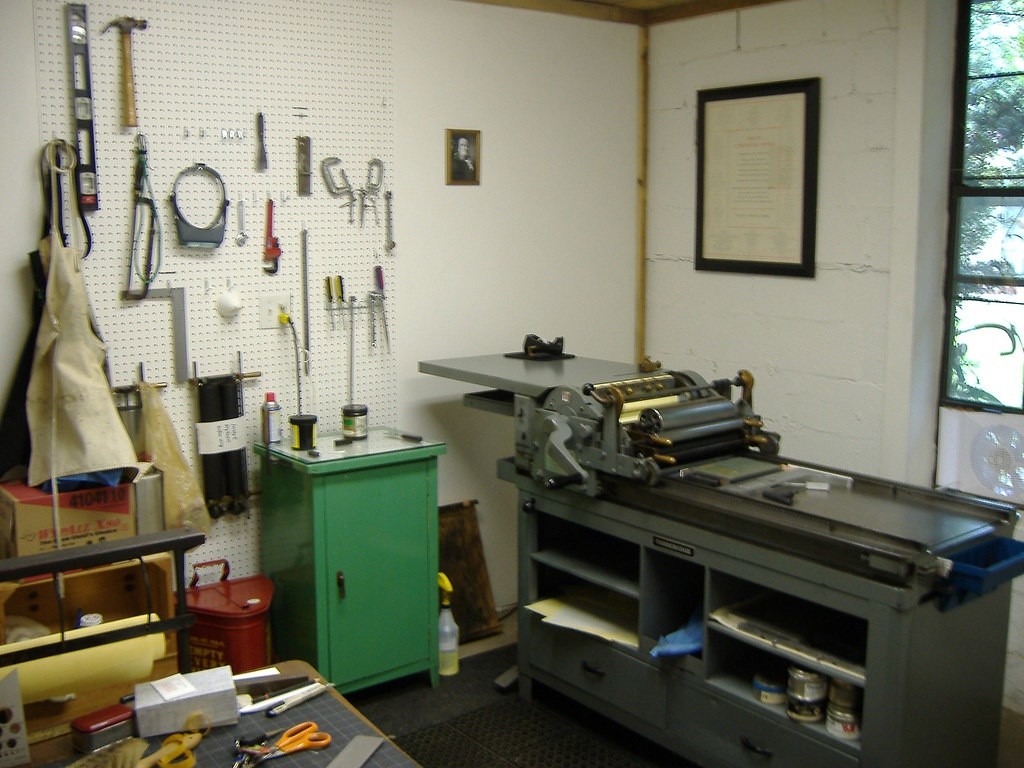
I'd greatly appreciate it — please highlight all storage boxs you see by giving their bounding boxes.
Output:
[1,472,135,584]
[0,551,179,748]
[135,666,238,737]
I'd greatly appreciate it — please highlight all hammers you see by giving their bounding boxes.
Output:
[102,16,147,127]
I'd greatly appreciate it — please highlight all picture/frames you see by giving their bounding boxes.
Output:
[444,128,481,186]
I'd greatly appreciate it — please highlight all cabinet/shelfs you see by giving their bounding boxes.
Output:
[497,455,1021,768]
[254,424,446,694]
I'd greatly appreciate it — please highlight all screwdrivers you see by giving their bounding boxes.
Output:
[335,275,346,330]
[325,276,335,331]
[375,265,390,354]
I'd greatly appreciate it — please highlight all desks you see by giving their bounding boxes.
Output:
[0,659,425,768]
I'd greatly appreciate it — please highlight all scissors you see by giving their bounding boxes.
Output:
[254,721,332,764]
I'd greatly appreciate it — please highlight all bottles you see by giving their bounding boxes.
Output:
[260,392,281,444]
